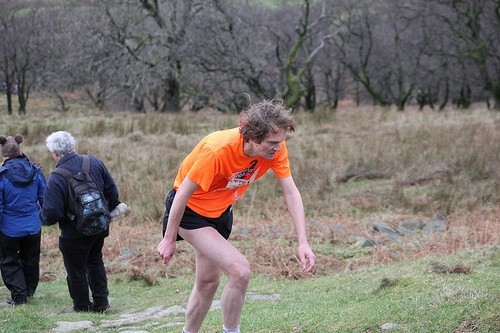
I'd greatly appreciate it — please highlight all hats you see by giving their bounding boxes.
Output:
[0,134,23,156]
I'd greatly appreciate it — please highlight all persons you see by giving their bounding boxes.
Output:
[157,98,316,333]
[39,130,121,316]
[0,134,46,308]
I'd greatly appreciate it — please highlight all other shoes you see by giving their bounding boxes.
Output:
[90,298,111,311]
[0,296,34,308]
[63,306,89,313]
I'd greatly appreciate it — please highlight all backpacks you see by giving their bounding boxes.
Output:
[50,154,110,243]
